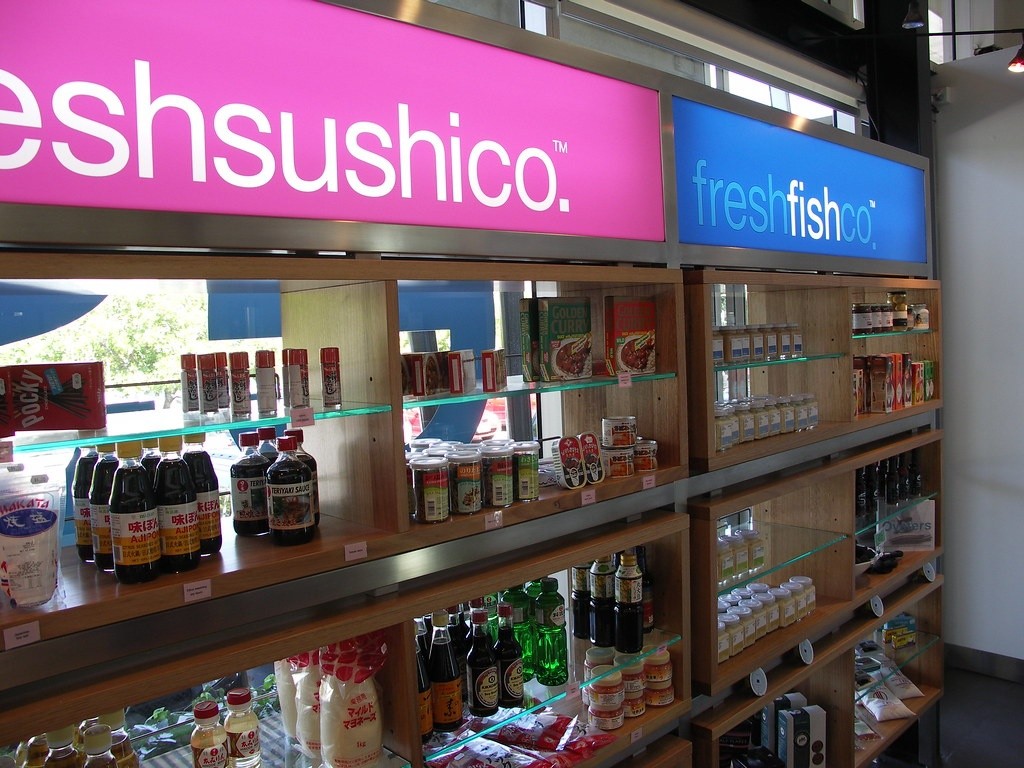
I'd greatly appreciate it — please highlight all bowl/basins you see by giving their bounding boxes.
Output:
[855,544,878,578]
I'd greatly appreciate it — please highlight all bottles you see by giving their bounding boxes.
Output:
[224,688,262,768]
[712,323,803,366]
[72,427,321,585]
[410,552,674,731]
[190,700,230,768]
[855,449,922,518]
[714,393,819,455]
[406,438,541,525]
[0,708,139,767]
[851,290,930,338]
[716,528,816,663]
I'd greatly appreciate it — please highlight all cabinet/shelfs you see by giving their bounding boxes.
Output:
[685,263,945,472]
[688,277,954,768]
[0,1,698,768]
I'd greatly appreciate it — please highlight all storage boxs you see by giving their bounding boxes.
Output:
[8,361,106,429]
[603,295,656,377]
[778,704,826,768]
[850,349,936,412]
[520,298,539,382]
[761,692,809,755]
[538,297,592,379]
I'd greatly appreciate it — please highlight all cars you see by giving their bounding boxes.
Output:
[403,391,536,442]
